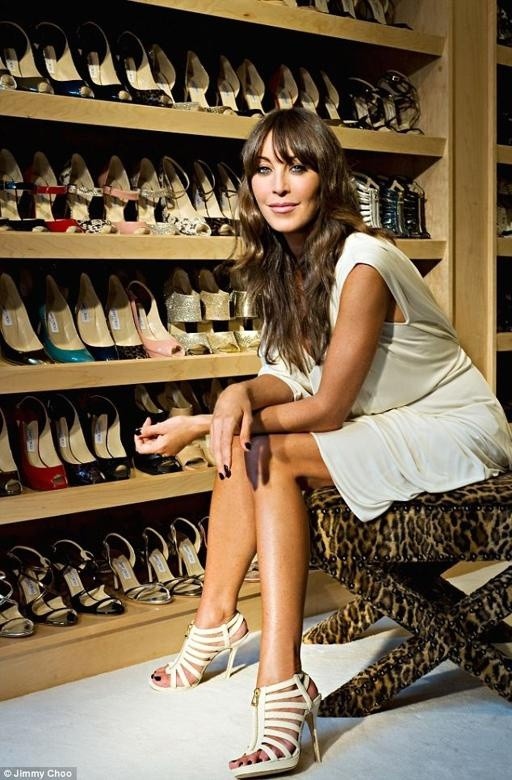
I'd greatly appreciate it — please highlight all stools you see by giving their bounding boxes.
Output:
[300,481,510,716]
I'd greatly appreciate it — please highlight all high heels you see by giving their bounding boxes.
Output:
[191,158,234,236]
[362,0,414,29]
[214,53,268,118]
[104,273,150,361]
[49,535,125,616]
[131,157,180,237]
[164,269,214,354]
[298,64,342,125]
[271,64,299,113]
[148,42,204,111]
[347,75,387,131]
[229,284,264,354]
[308,0,330,14]
[0,148,52,232]
[216,160,245,237]
[7,541,83,627]
[34,22,96,100]
[27,151,85,235]
[140,517,207,598]
[385,70,426,135]
[116,31,173,108]
[402,177,431,240]
[182,49,238,115]
[227,671,323,780]
[195,514,268,585]
[333,0,362,20]
[103,531,175,605]
[0,20,55,94]
[73,23,135,102]
[0,272,56,365]
[145,611,252,694]
[64,271,120,362]
[158,156,213,237]
[15,396,69,492]
[196,269,241,355]
[48,396,105,485]
[0,567,37,640]
[36,273,95,363]
[350,171,384,236]
[317,68,360,128]
[375,174,410,240]
[127,279,187,359]
[0,406,23,497]
[0,56,18,92]
[235,57,277,120]
[59,153,119,235]
[157,382,227,473]
[167,515,206,595]
[132,385,183,476]
[98,154,150,235]
[87,393,130,482]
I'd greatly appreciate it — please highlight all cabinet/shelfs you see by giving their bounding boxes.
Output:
[1,0,456,701]
[456,1,512,431]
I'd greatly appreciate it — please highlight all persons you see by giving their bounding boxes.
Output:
[134,106,512,780]
